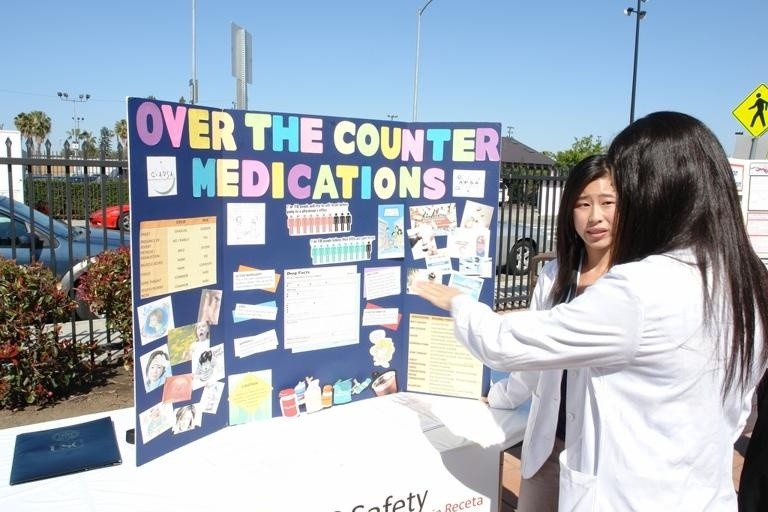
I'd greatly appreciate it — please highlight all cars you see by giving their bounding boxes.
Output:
[24,167,130,231]
[0,191,130,321]
[494,217,543,277]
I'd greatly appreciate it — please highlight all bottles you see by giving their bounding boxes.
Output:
[279,374,357,417]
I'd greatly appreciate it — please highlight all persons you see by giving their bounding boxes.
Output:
[146,351,170,386]
[175,405,196,430]
[476,236,487,257]
[485,154,619,512]
[384,224,403,250]
[201,291,222,324]
[198,350,214,381]
[416,110,768,512]
[182,323,209,358]
[147,310,165,332]
[36,200,46,212]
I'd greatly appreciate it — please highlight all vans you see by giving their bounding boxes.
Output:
[498,178,509,207]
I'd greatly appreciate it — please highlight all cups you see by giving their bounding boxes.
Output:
[370,368,398,397]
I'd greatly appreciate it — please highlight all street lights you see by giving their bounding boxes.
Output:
[56,88,92,177]
[385,113,399,120]
[71,115,85,128]
[620,0,649,127]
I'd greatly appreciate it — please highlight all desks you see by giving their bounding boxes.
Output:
[1,389,530,511]
